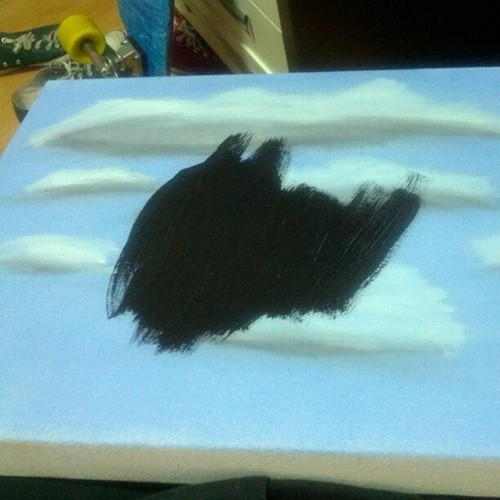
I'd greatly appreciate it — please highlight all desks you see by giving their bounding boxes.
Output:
[1,63,499,496]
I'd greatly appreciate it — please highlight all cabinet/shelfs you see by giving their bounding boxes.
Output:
[173,0,378,75]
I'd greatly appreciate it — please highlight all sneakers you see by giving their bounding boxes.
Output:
[12,29,132,126]
[169,14,229,75]
[0,22,71,75]
